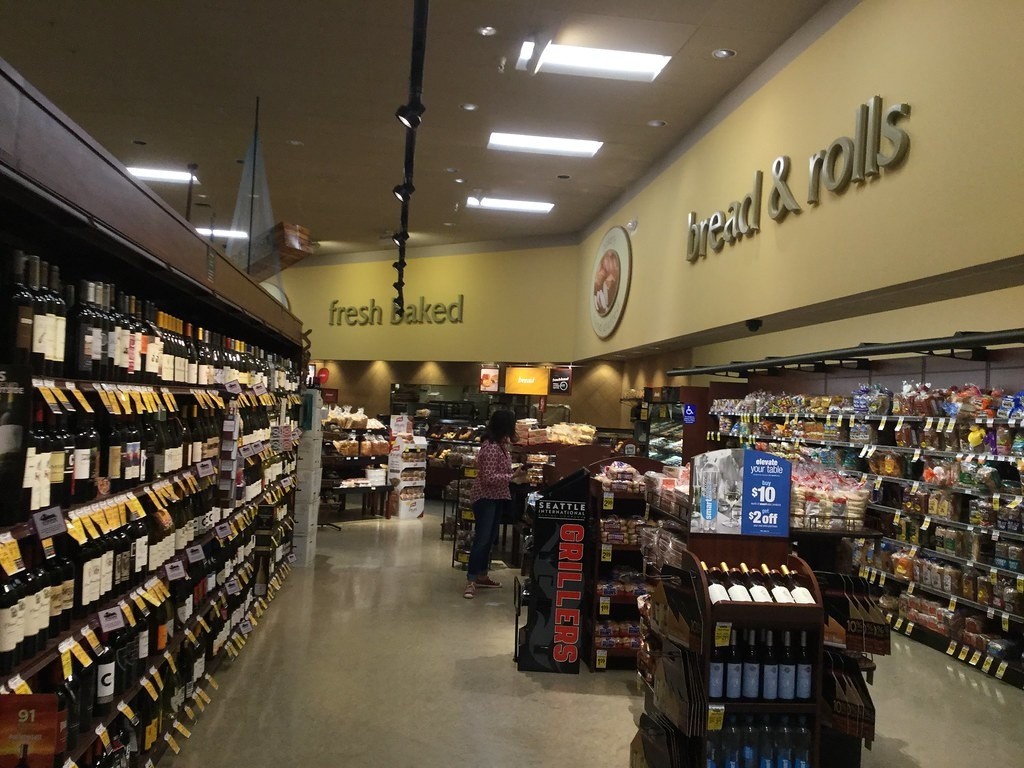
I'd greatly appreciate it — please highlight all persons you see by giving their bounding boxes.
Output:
[464,409,527,598]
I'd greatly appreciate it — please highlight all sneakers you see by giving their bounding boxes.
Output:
[463,582,476,598]
[473,577,502,588]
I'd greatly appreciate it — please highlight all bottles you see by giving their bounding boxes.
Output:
[740,563,773,602]
[22,398,226,521]
[707,715,811,768]
[720,562,753,602]
[761,564,795,604]
[10,248,197,387]
[779,565,816,604]
[763,630,778,703]
[779,627,811,703]
[198,328,301,395]
[0,382,24,527]
[236,470,246,507]
[228,406,235,420]
[700,560,730,604]
[245,449,297,596]
[0,474,258,768]
[741,627,766,703]
[231,397,301,446]
[708,628,741,702]
[699,457,719,531]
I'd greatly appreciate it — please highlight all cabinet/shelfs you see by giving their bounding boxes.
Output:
[405,325,1024,768]
[0,60,303,768]
[318,426,394,516]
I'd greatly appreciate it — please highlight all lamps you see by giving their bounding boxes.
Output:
[392,102,425,317]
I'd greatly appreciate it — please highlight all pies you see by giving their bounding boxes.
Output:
[790,487,868,530]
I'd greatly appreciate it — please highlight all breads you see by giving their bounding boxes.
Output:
[481,374,499,386]
[594,466,656,651]
[509,420,596,446]
[329,413,389,456]
[395,433,424,501]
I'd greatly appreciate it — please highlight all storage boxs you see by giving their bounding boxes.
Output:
[293,389,322,567]
